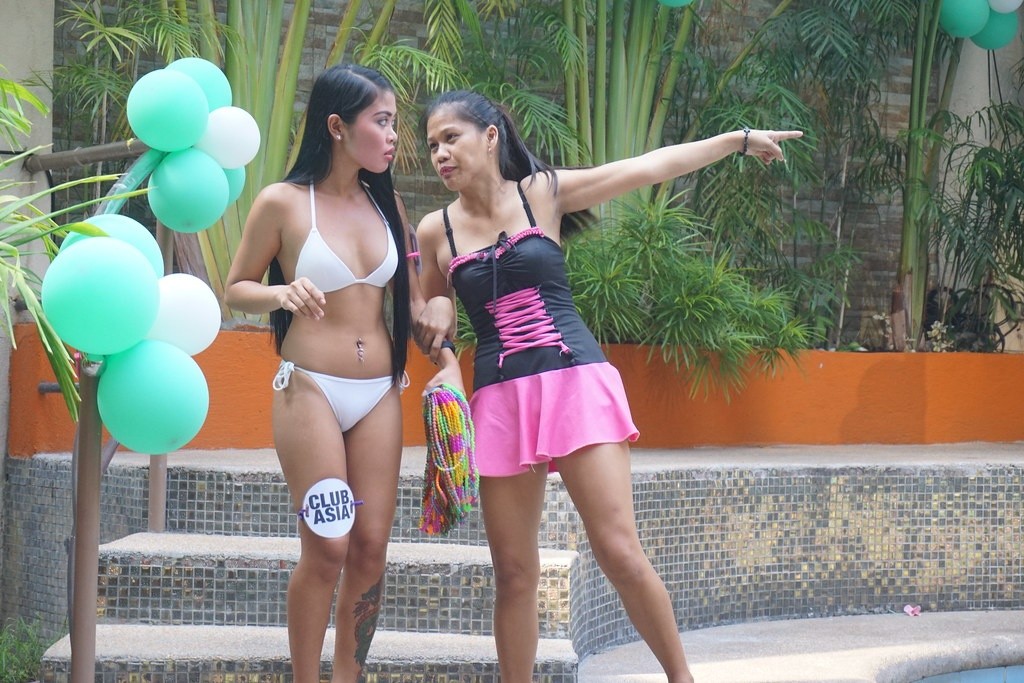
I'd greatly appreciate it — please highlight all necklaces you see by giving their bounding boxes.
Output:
[418,383,480,536]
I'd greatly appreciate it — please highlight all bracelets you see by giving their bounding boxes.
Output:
[738,126,750,157]
[441,341,456,355]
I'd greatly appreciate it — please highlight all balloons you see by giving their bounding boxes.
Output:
[656,0,694,8]
[126,57,261,234]
[41,214,222,455]
[938,0,1024,51]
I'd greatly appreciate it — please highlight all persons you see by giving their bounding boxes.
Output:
[416,88,804,683]
[223,62,467,683]
